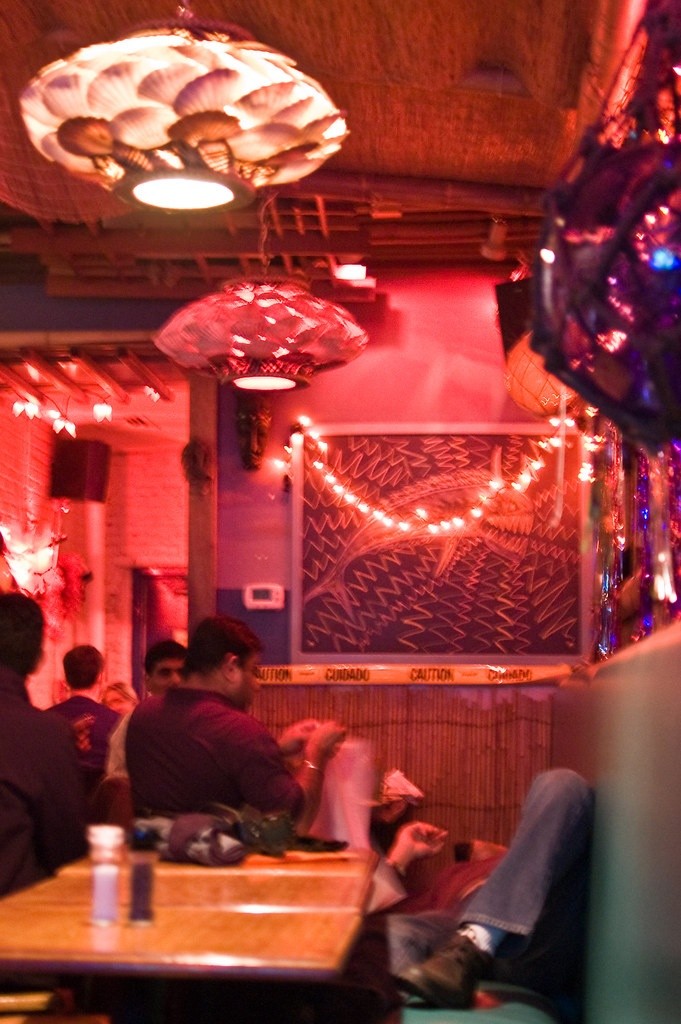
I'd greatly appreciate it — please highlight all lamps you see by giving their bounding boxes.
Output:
[530,0,681,455]
[20,1,352,215]
[150,253,370,393]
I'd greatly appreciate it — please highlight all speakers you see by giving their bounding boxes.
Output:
[51,438,110,503]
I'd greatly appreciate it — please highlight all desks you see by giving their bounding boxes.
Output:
[1,849,378,1024]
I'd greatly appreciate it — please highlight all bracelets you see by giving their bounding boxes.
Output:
[302,757,327,778]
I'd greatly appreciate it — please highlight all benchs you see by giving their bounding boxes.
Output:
[369,626,681,1024]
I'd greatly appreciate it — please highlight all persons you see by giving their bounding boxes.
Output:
[0,596,593,1012]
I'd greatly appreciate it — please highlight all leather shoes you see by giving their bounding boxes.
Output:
[392,929,492,1008]
[310,980,388,1024]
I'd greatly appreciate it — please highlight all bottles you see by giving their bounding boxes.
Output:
[90,866,119,925]
[129,860,156,924]
[85,826,124,863]
